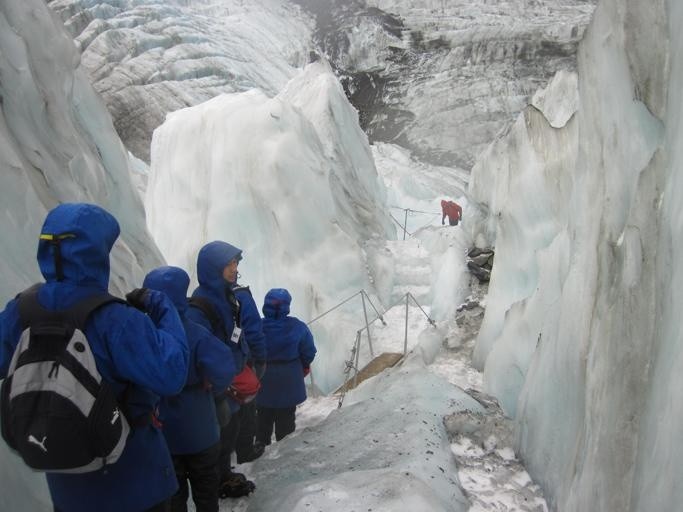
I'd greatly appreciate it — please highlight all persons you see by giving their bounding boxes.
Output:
[141,267,240,512]
[0,201,191,512]
[439,199,462,226]
[251,288,318,448]
[186,239,259,502]
[231,281,267,465]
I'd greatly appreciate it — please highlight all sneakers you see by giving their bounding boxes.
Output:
[237,443,266,463]
[217,473,256,499]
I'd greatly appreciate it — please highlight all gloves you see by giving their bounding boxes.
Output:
[126,288,148,310]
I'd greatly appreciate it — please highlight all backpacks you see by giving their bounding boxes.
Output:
[0,283,132,478]
